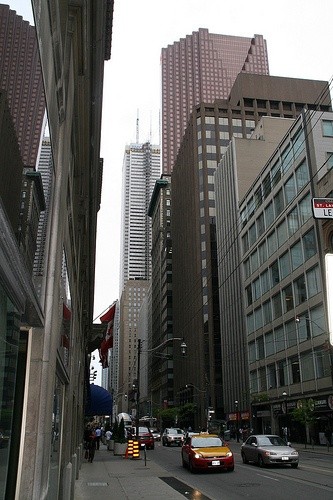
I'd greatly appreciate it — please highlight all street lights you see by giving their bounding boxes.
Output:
[235,400,240,442]
[185,384,215,431]
[294,316,333,376]
[282,391,289,442]
[136,337,187,441]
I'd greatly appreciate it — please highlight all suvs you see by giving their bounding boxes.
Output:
[161,427,185,446]
[132,426,155,449]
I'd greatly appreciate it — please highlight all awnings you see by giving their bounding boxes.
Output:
[87,383,113,417]
[84,324,107,354]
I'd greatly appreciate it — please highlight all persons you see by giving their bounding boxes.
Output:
[284,426,290,442]
[238,427,243,441]
[95,427,101,451]
[87,431,96,463]
[105,428,112,450]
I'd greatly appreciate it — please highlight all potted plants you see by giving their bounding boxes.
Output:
[101,416,128,456]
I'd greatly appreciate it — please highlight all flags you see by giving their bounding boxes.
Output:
[99,303,116,369]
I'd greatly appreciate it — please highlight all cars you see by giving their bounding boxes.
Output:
[180,433,234,474]
[149,428,162,441]
[240,435,299,468]
